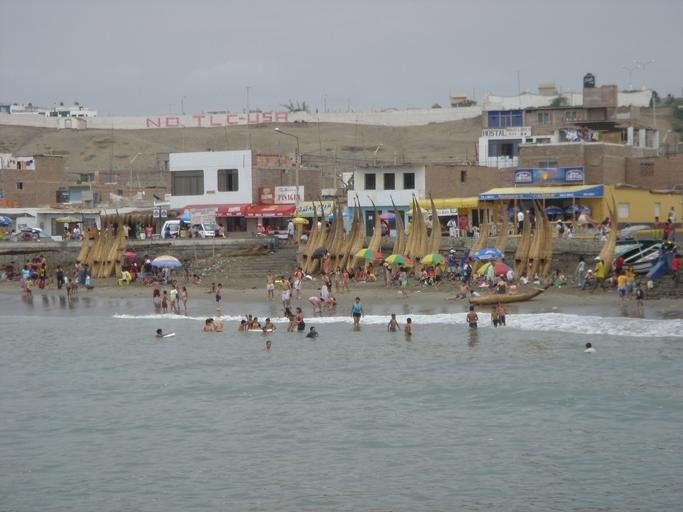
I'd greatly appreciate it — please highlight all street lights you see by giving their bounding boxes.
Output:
[274,127,301,219]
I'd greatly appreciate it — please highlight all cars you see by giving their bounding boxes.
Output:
[199,223,226,238]
[12,228,53,241]
[161,221,192,239]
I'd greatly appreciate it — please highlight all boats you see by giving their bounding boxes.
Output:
[75,209,126,277]
[299,193,553,276]
[593,190,673,277]
[470,283,553,303]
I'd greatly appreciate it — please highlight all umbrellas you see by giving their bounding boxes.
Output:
[0,214,16,230]
[175,213,195,228]
[291,206,429,237]
[55,216,84,231]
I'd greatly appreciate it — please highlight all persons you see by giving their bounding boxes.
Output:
[256,219,295,247]
[0,254,95,295]
[0,224,22,242]
[117,246,682,352]
[446,215,469,238]
[64,219,225,240]
[517,207,676,241]
